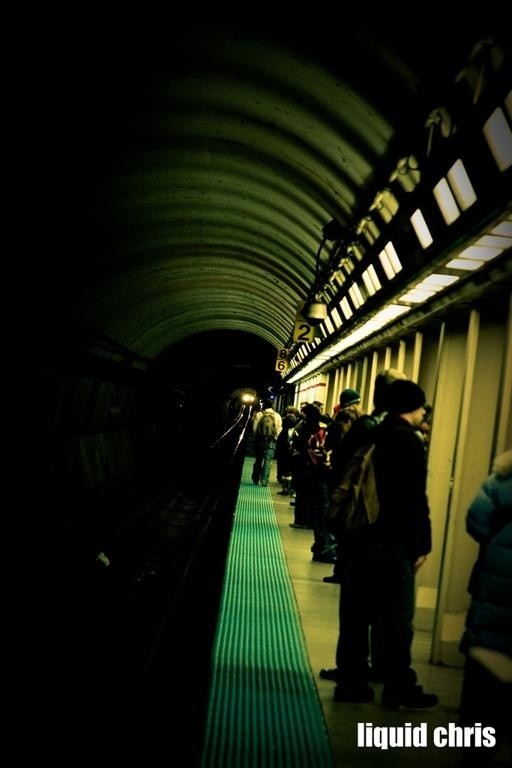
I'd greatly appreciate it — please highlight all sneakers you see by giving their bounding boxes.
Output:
[253,480,268,486]
[318,663,440,712]
[277,478,338,583]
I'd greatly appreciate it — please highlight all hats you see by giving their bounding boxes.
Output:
[381,380,426,414]
[339,389,361,408]
[373,368,408,407]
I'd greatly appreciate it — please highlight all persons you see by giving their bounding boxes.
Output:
[441,442,512,715]
[249,370,440,714]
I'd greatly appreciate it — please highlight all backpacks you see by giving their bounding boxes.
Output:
[256,411,277,443]
[323,445,380,541]
[309,429,328,467]
